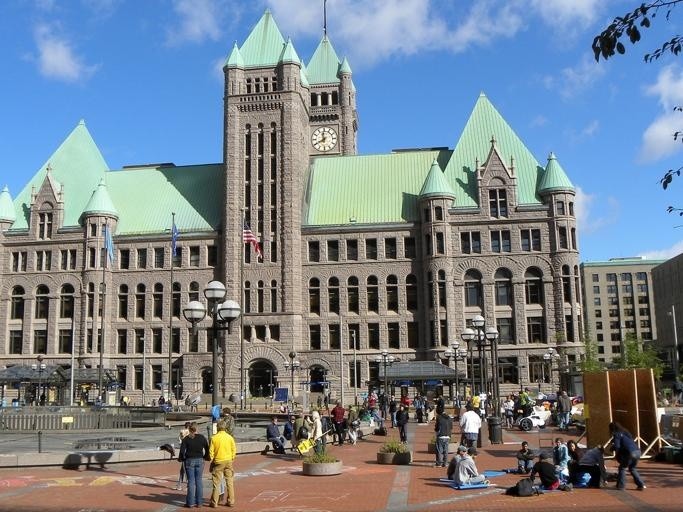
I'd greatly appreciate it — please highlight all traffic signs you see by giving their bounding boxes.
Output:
[283,351,300,411]
[443,340,468,422]
[181,279,240,436]
[374,349,395,420]
[460,315,499,448]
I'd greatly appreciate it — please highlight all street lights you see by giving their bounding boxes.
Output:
[300,367,314,414]
[666,305,680,378]
[103,368,122,391]
[152,370,167,403]
[237,366,254,410]
[31,355,45,405]
[139,337,145,406]
[265,368,277,407]
[318,368,330,399]
[515,365,525,394]
[542,347,560,394]
[172,368,186,406]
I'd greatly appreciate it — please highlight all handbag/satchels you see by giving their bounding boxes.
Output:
[515,478,538,496]
[351,418,361,429]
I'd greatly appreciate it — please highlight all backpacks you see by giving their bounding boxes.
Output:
[298,427,308,439]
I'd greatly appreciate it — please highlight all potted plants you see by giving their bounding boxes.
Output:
[376,439,413,464]
[428,434,459,453]
[303,451,342,475]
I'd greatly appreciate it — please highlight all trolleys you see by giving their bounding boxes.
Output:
[538,428,554,451]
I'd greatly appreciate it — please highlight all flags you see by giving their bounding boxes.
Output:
[106,224,113,266]
[243,218,262,260]
[172,222,179,257]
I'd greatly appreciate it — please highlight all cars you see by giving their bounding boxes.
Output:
[511,408,546,433]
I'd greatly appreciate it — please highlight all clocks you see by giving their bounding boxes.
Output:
[311,126,338,152]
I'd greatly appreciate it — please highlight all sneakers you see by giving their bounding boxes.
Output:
[637,485,646,489]
[184,504,193,508]
[172,485,182,490]
[197,504,204,508]
[433,465,442,468]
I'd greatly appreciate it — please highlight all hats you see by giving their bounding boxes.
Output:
[458,446,467,452]
[468,448,480,453]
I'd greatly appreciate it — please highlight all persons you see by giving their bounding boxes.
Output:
[158,396,165,405]
[229,393,233,402]
[185,395,193,405]
[176,408,236,508]
[655,376,683,438]
[258,385,263,397]
[266,391,648,492]
[40,393,46,406]
